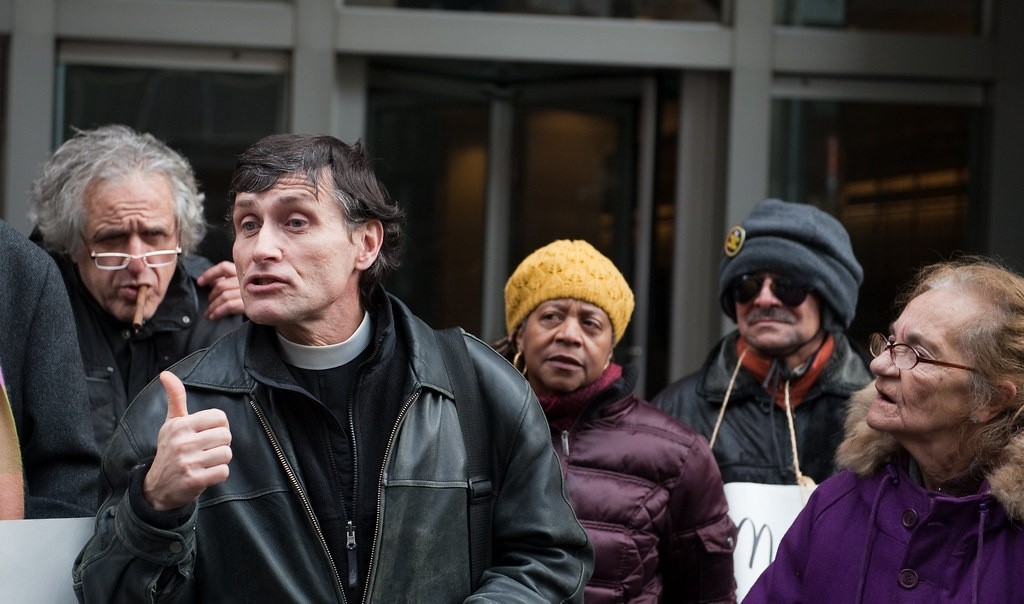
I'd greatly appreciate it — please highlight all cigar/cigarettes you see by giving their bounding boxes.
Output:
[131,284,149,330]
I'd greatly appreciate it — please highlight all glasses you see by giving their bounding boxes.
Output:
[732,273,813,307]
[870,333,987,377]
[76,227,183,270]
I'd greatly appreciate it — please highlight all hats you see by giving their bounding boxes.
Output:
[505,240,635,348]
[716,199,864,331]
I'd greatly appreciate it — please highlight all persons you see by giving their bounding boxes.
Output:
[648,197,876,487]
[1,217,102,519]
[69,130,601,604]
[487,236,740,604]
[737,243,1023,604]
[26,122,246,454]
